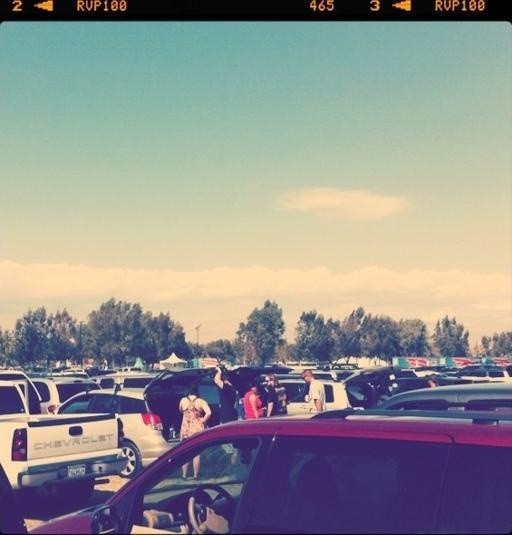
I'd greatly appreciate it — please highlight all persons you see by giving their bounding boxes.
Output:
[264,373,289,417]
[243,383,264,420]
[214,366,239,481]
[301,369,326,412]
[178,384,212,480]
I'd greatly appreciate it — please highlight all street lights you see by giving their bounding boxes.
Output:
[195,324,202,367]
